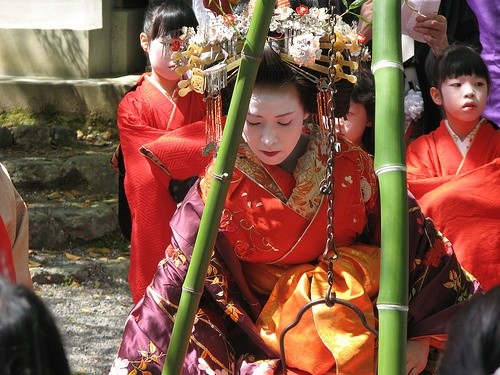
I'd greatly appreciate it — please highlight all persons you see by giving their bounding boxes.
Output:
[0,161,71,375]
[109,0,500,375]
